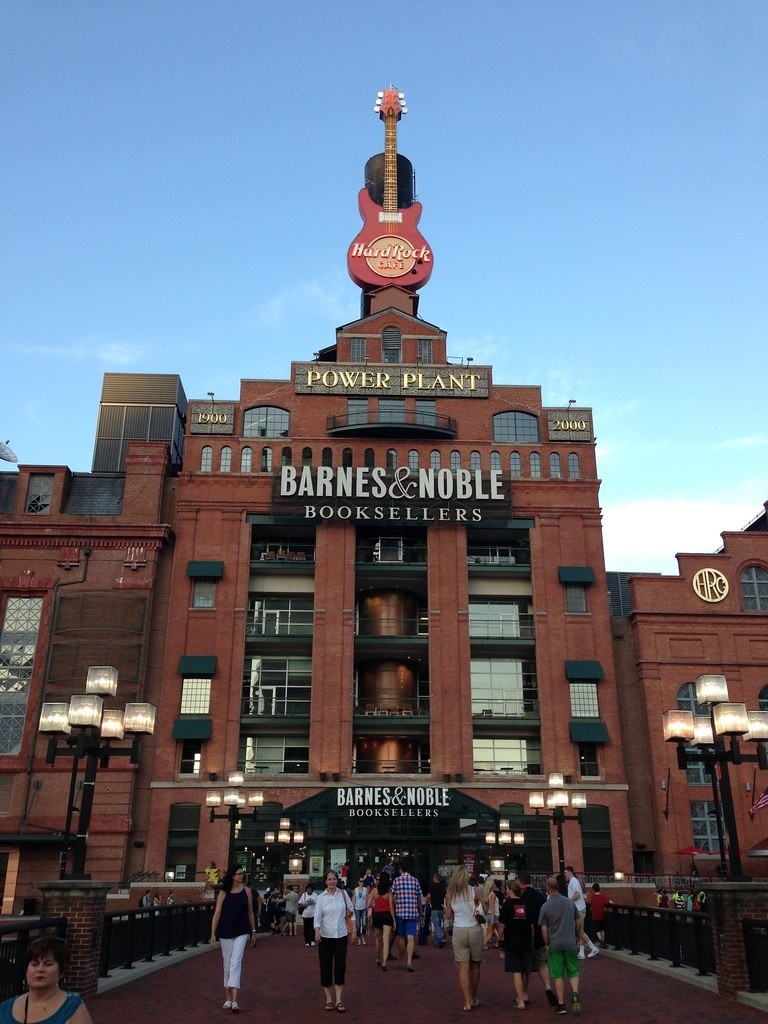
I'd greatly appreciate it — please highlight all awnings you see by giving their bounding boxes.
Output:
[559,566,596,582]
[565,661,603,678]
[177,655,217,674]
[569,722,609,743]
[172,720,212,740]
[186,561,224,579]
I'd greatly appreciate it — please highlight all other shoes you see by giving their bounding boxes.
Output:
[232,1001,239,1013]
[223,1001,232,1009]
[406,964,416,972]
[311,941,315,946]
[555,1004,567,1014]
[305,943,310,947]
[375,959,381,967]
[546,989,557,1006]
[572,992,581,1014]
[387,953,397,961]
[382,964,390,971]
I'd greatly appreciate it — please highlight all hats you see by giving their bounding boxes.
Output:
[168,890,174,895]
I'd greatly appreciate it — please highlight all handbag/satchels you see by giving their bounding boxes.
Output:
[474,887,487,923]
[346,907,353,935]
[298,906,305,915]
[367,907,372,919]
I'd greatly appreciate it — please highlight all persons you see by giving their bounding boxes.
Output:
[153,891,162,916]
[657,888,708,912]
[338,858,453,972]
[445,864,485,1011]
[467,866,613,1016]
[165,891,176,914]
[313,870,357,1012]
[0,936,93,1024]
[248,882,319,947]
[142,890,153,917]
[210,863,257,1011]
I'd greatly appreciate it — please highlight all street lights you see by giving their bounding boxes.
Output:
[486,819,525,880]
[662,674,768,878]
[264,817,304,891]
[38,664,158,880]
[205,771,264,878]
[529,772,587,874]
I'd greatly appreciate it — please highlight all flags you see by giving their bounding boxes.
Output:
[749,788,768,815]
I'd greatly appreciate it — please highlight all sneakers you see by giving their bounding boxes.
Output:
[587,948,600,957]
[577,952,585,960]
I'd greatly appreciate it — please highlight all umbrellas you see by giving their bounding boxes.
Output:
[708,845,746,856]
[671,845,706,866]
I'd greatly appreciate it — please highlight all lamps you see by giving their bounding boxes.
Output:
[364,355,371,367]
[134,839,143,848]
[319,772,326,781]
[455,773,462,782]
[417,355,423,369]
[467,358,473,368]
[209,772,217,781]
[636,842,646,849]
[313,352,321,367]
[567,400,576,410]
[565,775,572,782]
[333,773,339,781]
[444,774,451,783]
[207,392,215,405]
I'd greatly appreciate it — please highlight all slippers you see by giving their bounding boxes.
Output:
[325,1002,334,1011]
[461,1005,472,1012]
[335,1002,345,1012]
[470,999,480,1007]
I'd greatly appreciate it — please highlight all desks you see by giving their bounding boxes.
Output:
[253,765,270,774]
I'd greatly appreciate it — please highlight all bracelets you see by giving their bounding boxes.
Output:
[352,930,356,932]
[252,930,256,932]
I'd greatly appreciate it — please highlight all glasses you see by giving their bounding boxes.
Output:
[359,881,364,883]
[235,872,244,875]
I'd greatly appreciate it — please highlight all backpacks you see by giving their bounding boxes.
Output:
[138,896,147,907]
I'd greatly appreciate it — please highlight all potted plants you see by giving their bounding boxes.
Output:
[475,557,481,563]
[414,543,427,562]
[516,536,530,563]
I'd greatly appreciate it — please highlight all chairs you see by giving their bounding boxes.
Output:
[482,709,496,717]
[365,703,413,716]
[264,551,306,561]
